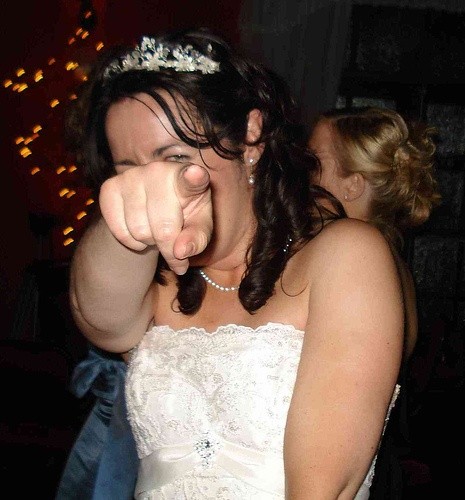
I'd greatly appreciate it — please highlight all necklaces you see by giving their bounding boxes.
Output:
[199,269,240,292]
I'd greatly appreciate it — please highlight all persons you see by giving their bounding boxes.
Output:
[308,108,441,365]
[56,346,140,500]
[69,26,403,500]
